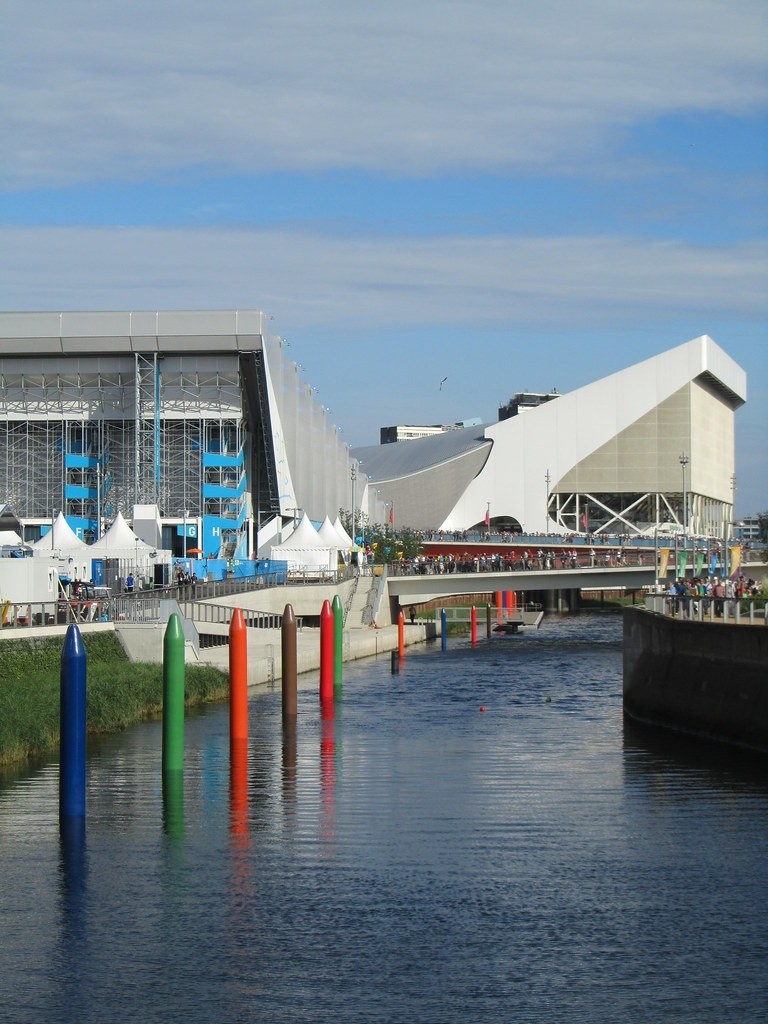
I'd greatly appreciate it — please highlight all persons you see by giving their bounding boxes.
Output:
[74,579,79,595]
[174,559,198,594]
[126,573,133,596]
[393,529,751,574]
[90,579,92,583]
[666,576,763,618]
[409,605,417,624]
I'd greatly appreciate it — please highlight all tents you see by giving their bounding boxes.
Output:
[270,511,367,578]
[0,510,172,587]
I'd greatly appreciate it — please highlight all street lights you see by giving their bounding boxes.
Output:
[675,532,688,583]
[286,507,304,531]
[679,451,689,549]
[104,555,108,587]
[723,520,745,577]
[135,538,139,569]
[655,528,669,594]
[351,462,357,549]
[545,469,551,533]
[52,509,57,550]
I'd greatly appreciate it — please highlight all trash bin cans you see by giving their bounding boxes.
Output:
[226,571,233,579]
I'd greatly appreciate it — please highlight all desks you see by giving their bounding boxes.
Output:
[83,587,112,599]
